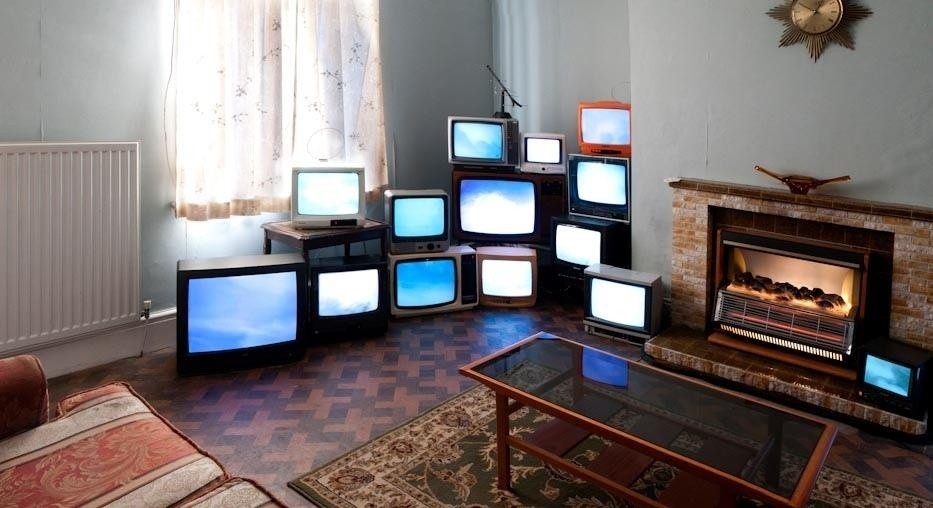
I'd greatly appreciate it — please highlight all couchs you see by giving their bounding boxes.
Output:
[0,347,315,507]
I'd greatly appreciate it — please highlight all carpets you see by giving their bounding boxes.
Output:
[287,356,929,504]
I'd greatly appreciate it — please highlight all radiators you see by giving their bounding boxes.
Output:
[0,139,149,347]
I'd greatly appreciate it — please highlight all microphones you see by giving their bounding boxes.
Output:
[486,64,521,118]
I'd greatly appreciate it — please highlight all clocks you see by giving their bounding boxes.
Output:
[764,0,873,61]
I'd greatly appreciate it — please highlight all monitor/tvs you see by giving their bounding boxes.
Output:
[451,168,566,245]
[856,337,933,418]
[177,253,307,376]
[307,254,388,347]
[291,167,367,231]
[519,133,566,175]
[477,246,538,308]
[388,246,479,318]
[581,347,630,388]
[554,100,661,334]
[384,189,451,255]
[447,115,519,167]
